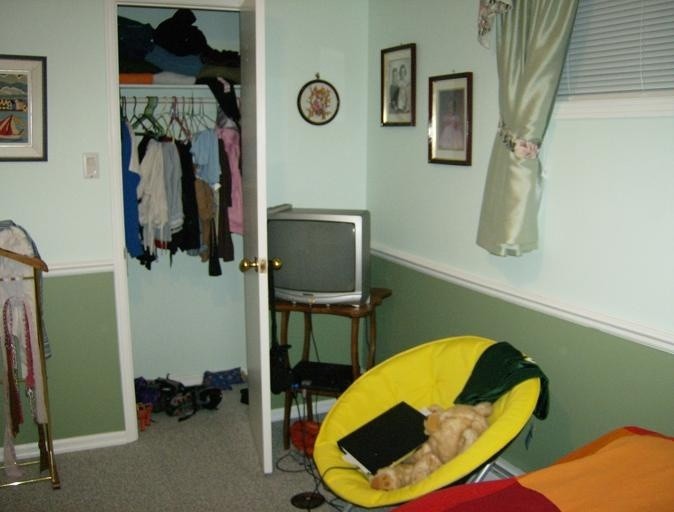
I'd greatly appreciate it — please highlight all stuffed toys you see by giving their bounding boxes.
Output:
[367,400,495,494]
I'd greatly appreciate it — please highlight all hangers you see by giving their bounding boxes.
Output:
[121,94,218,144]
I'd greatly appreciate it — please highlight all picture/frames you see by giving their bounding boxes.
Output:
[429,72,472,165]
[0,55,47,162]
[382,43,415,125]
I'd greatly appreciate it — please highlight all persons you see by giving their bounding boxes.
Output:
[398,64,412,112]
[438,99,465,150]
[388,67,401,113]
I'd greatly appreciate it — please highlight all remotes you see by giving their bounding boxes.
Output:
[267,204,291,215]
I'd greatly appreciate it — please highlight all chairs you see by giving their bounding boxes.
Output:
[313,335,542,512]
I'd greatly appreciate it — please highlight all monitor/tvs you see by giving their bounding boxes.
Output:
[267,209,372,307]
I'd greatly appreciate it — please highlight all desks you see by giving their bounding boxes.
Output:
[269,288,391,450]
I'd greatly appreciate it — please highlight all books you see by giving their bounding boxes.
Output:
[334,401,431,477]
